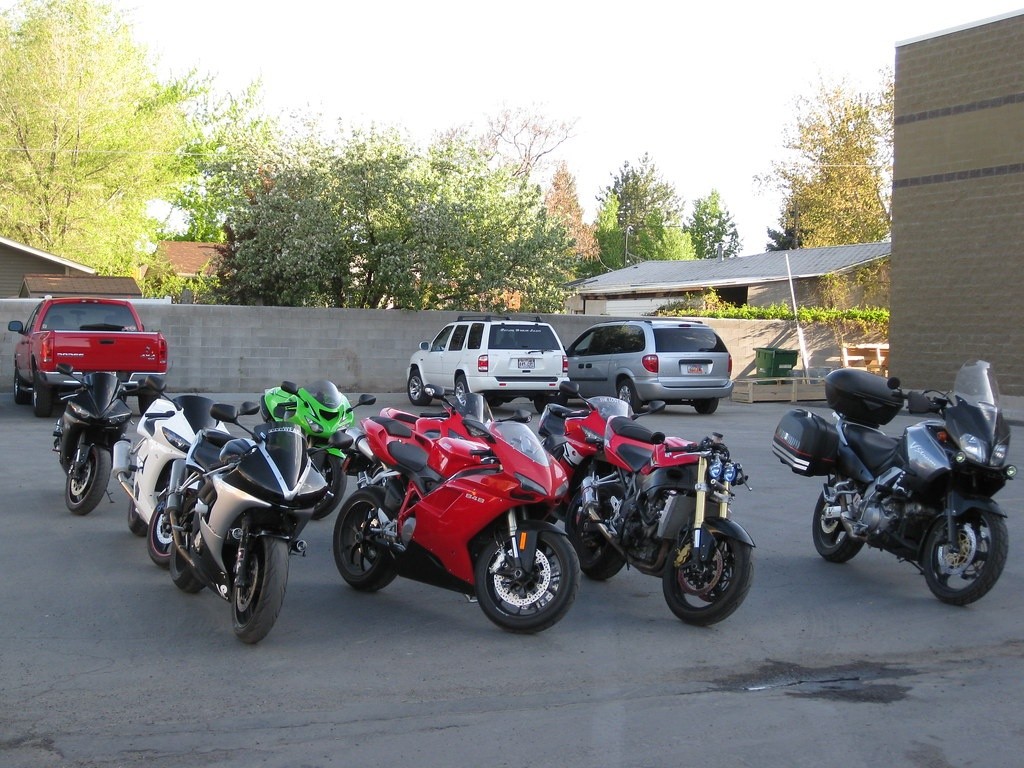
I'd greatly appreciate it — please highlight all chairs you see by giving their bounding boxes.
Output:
[104,315,123,326]
[49,315,64,326]
[500,335,514,345]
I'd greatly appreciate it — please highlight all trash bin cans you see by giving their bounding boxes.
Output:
[753,345,799,384]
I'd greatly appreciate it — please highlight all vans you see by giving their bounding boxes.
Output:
[563,318,734,413]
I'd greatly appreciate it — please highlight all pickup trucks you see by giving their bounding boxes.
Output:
[7,297,169,419]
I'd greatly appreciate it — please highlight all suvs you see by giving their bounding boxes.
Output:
[405,314,570,413]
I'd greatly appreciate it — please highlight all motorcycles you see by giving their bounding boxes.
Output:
[168,402,355,644]
[342,379,533,491]
[566,414,755,626]
[532,381,668,535]
[770,360,1017,607]
[262,379,376,519]
[49,362,161,516]
[112,371,261,562]
[329,414,582,634]
